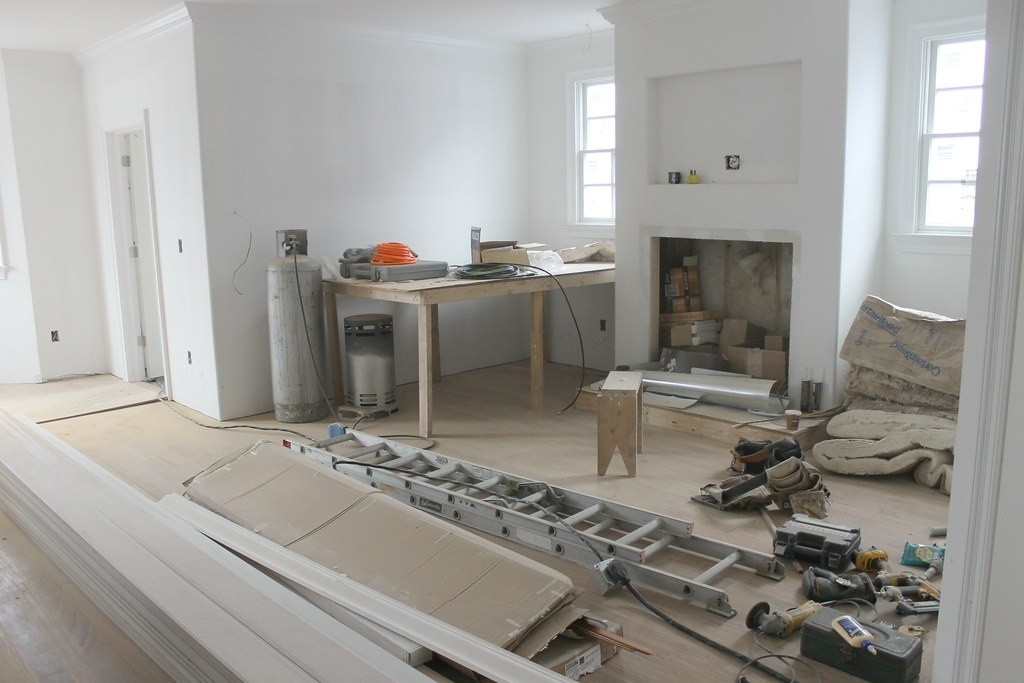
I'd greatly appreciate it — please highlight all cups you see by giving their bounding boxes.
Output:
[669,172,681,184]
[785,410,801,430]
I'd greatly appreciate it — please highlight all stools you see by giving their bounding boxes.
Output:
[597,371,643,477]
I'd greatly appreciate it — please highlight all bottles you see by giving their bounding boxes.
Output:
[831,615,877,655]
[688,170,700,183]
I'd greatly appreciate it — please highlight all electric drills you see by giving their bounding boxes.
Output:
[851,545,908,588]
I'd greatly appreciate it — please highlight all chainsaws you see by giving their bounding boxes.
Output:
[744,595,824,640]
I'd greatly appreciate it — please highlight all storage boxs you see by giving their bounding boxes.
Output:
[801,606,924,683]
[470,227,547,266]
[659,256,788,395]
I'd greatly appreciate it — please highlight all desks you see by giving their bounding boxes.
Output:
[320,260,616,439]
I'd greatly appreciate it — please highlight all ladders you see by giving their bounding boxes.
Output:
[281,422,786,620]
